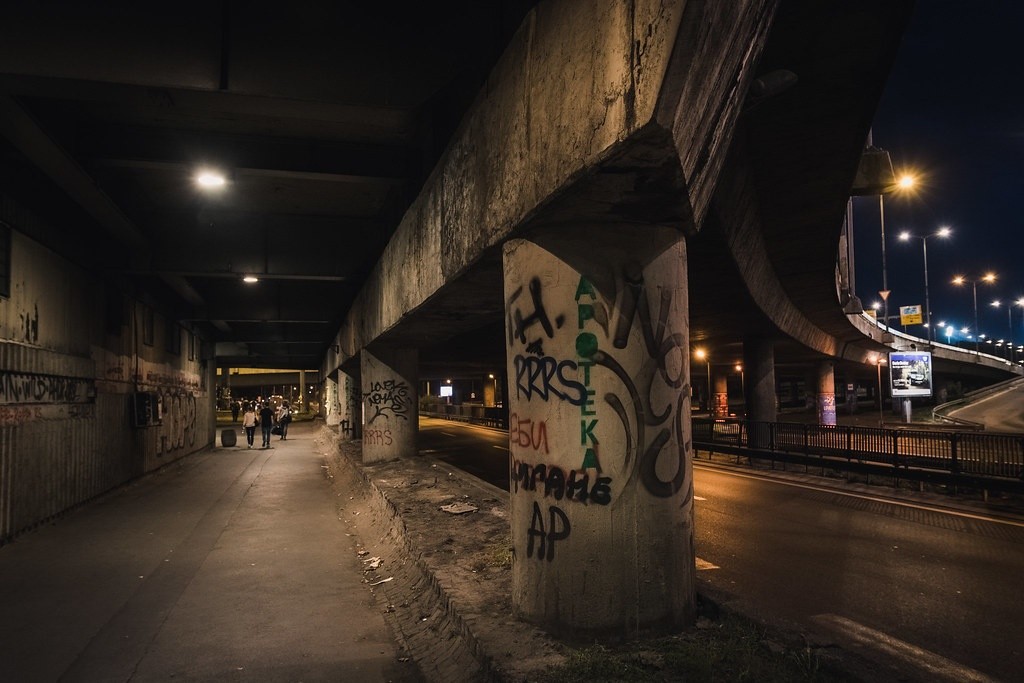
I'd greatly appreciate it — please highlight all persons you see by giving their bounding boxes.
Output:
[252,401,256,410]
[241,400,251,412]
[243,407,258,448]
[259,402,276,448]
[230,399,241,421]
[279,401,294,441]
[849,396,855,415]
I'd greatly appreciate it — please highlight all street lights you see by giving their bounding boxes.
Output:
[880,165,1024,365]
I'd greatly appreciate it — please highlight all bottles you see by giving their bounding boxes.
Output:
[242,426,245,434]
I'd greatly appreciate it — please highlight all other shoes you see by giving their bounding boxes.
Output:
[248,444,253,449]
[262,439,266,447]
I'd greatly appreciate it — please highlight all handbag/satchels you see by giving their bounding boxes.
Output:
[271,425,283,435]
[287,414,292,423]
[280,417,289,426]
[254,411,260,427]
